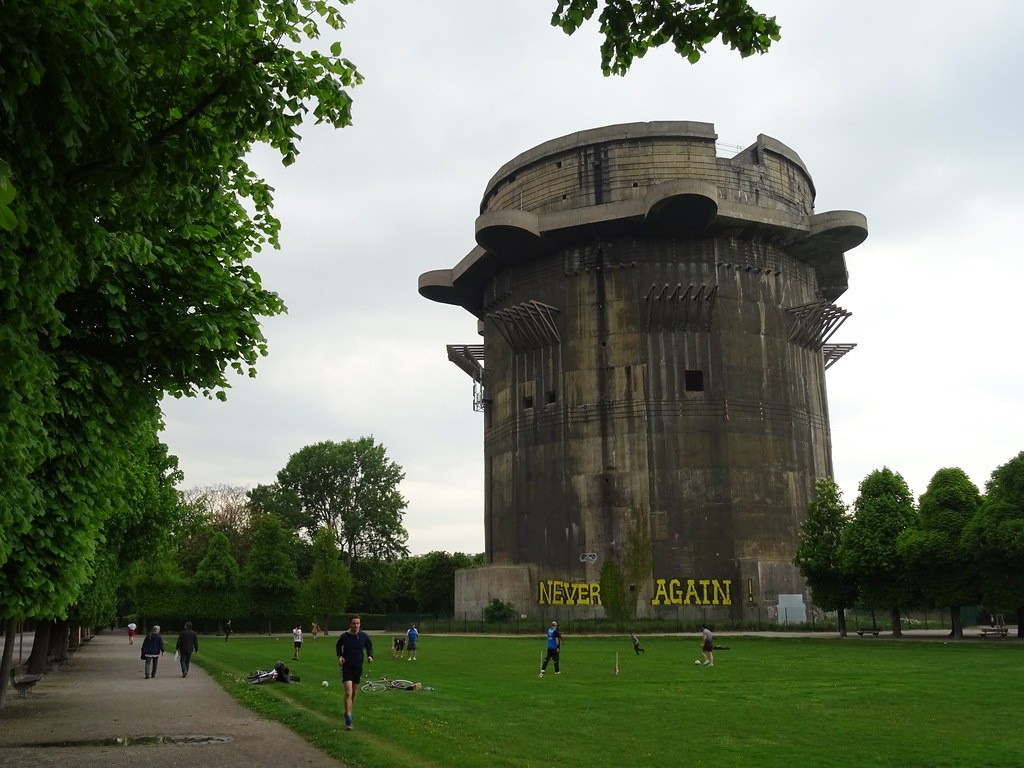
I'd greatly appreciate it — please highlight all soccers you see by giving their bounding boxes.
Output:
[322,680,330,688]
[694,659,702,665]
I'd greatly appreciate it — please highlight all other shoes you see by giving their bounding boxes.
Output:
[704,660,710,666]
[408,657,411,660]
[345,715,353,730]
[709,663,713,666]
[152,674,155,678]
[182,671,187,678]
[146,674,149,679]
[413,657,416,660]
[555,671,561,674]
[542,670,546,674]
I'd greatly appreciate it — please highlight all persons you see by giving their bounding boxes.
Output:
[630,632,645,655]
[291,623,303,661]
[311,622,320,640]
[335,613,374,729]
[176,621,199,678]
[224,619,232,642]
[391,637,407,658]
[541,621,562,674]
[700,624,714,667]
[141,625,164,679]
[404,623,419,661]
[127,620,137,645]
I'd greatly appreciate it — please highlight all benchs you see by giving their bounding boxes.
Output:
[857,628,883,639]
[10,665,44,698]
[66,635,96,657]
[978,629,1008,640]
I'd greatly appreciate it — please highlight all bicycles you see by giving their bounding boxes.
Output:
[359,674,414,695]
[247,660,279,685]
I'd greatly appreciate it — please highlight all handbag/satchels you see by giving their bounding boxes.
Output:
[141,649,148,659]
[276,662,291,682]
[174,650,180,662]
